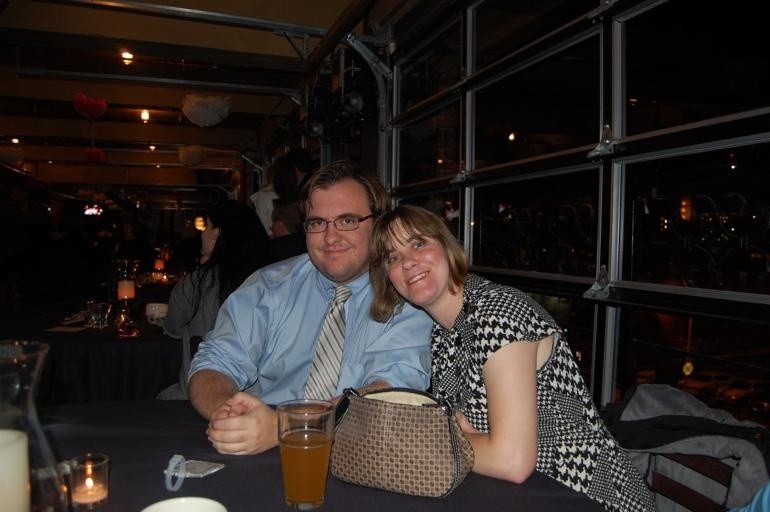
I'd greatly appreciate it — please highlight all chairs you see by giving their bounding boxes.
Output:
[641,384,769,512]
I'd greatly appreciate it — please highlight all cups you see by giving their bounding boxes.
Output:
[69,452,109,510]
[276,398,337,510]
[87,300,112,327]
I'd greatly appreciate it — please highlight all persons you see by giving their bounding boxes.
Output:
[185,161,435,456]
[369,205,659,512]
[153,199,279,400]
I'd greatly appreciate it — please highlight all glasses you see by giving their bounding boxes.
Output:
[303,213,376,234]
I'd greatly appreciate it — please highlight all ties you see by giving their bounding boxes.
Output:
[302,286,353,403]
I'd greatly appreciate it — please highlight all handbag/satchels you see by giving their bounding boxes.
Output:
[323,386,476,500]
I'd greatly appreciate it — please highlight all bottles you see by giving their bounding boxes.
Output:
[115,260,138,316]
[0,339,69,512]
